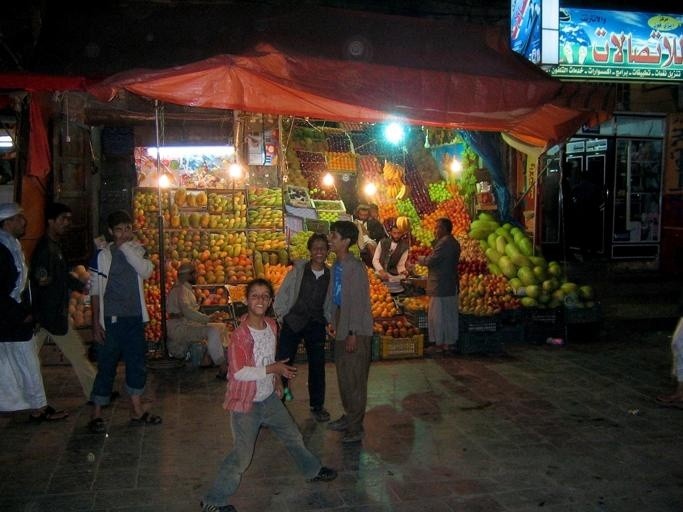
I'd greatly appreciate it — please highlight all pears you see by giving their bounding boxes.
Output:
[381,318,421,338]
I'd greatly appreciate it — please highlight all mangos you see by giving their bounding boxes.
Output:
[170,187,287,284]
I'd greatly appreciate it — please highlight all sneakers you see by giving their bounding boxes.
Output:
[307,402,367,446]
[198,499,238,512]
[303,466,340,485]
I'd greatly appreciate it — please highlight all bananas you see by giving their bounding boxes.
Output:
[383,161,409,200]
[422,195,471,236]
[397,216,411,232]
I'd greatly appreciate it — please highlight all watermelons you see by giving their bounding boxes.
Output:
[469,212,597,310]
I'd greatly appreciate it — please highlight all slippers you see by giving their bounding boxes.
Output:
[130,410,164,425]
[90,416,106,432]
[28,403,71,425]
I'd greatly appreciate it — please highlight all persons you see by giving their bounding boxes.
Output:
[657,317,683,404]
[199,278,338,512]
[322,220,374,443]
[363,204,387,244]
[273,234,331,421]
[372,226,409,293]
[30,203,121,407]
[86,209,162,431]
[416,218,461,356]
[1,202,70,423]
[353,205,377,253]
[167,264,230,382]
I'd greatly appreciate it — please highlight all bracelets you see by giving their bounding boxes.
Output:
[348,329,357,336]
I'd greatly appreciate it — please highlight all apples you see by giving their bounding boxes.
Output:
[458,257,520,316]
[130,191,177,342]
[194,287,226,305]
[66,265,93,327]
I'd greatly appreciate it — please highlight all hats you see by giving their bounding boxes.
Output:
[0,203,24,221]
